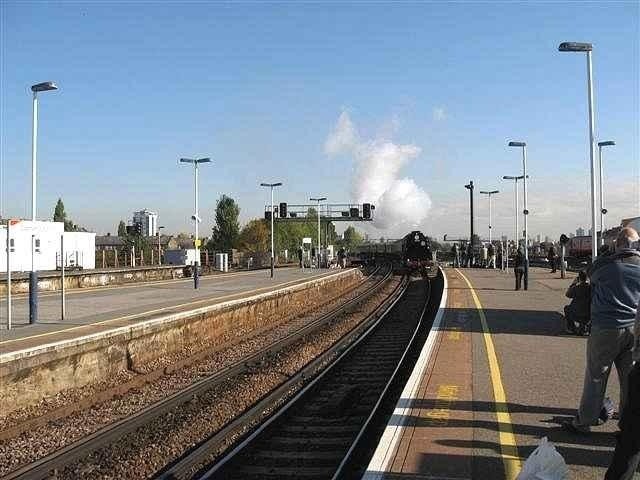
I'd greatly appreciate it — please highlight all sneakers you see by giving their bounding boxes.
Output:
[564,421,576,433]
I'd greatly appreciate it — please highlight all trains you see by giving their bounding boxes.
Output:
[346,230,433,277]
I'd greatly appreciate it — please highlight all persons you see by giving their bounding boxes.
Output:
[563,227,640,480]
[295,245,305,268]
[311,245,316,259]
[337,245,347,269]
[547,247,557,273]
[451,242,517,271]
[510,248,525,291]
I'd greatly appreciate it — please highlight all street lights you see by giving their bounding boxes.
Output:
[179,157,213,289]
[558,42,617,264]
[479,142,530,290]
[260,182,283,279]
[309,197,327,269]
[29,80,59,324]
